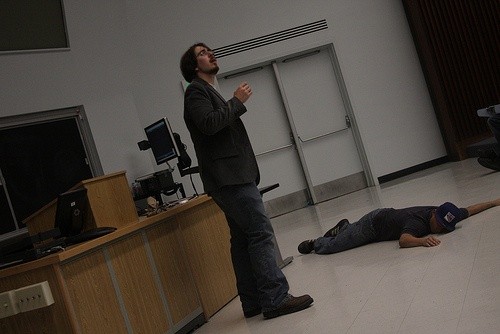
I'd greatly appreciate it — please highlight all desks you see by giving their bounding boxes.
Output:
[0,192,238,334]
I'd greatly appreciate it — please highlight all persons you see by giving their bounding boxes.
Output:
[179,42,314,321]
[298,197,500,256]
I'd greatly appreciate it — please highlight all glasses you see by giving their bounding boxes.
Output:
[195,48,214,59]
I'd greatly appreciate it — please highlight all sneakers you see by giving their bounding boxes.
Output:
[298,238,318,255]
[243,298,267,317]
[323,219,349,238]
[264,295,314,319]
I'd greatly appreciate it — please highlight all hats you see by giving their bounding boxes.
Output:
[436,203,460,230]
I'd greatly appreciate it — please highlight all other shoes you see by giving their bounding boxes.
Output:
[478,156,500,172]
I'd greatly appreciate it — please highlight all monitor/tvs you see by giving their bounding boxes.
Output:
[144,117,180,165]
[53,186,87,240]
